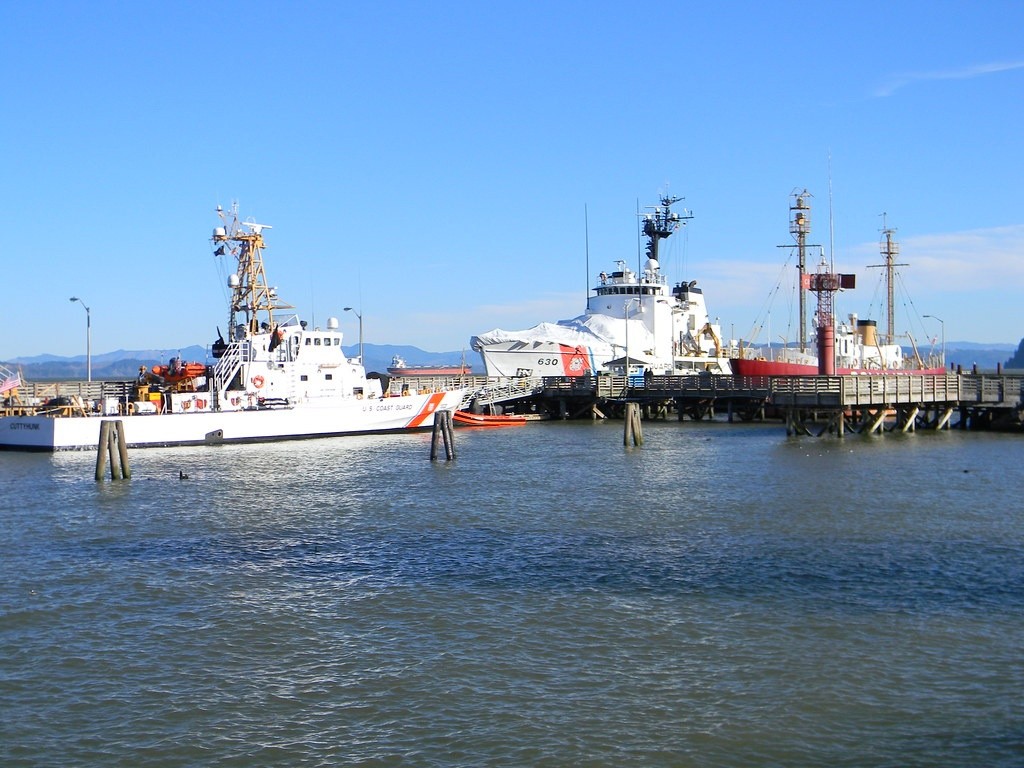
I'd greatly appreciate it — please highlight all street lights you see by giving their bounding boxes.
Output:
[921,315,944,352]
[344,308,363,358]
[70,296,91,379]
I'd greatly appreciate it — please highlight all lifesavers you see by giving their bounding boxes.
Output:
[426,388,431,394]
[384,392,390,398]
[435,387,441,393]
[403,390,408,396]
[254,376,265,388]
[356,393,363,399]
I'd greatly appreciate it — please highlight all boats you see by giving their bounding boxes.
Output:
[387,355,471,376]
[472,194,738,387]
[0,193,464,449]
[728,187,946,388]
